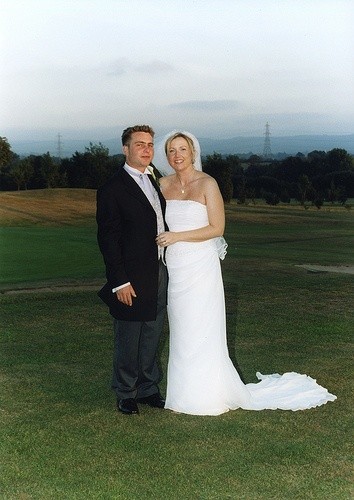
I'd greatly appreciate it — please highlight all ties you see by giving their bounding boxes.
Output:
[141,173,156,205]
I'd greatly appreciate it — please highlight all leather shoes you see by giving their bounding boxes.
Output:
[138,392,166,408]
[117,397,139,415]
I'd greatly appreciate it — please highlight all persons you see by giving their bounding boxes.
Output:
[152,129,246,416]
[96,124,170,413]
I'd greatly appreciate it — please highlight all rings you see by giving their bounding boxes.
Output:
[165,241,167,243]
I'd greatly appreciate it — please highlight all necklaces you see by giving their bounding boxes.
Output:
[182,189,185,193]
